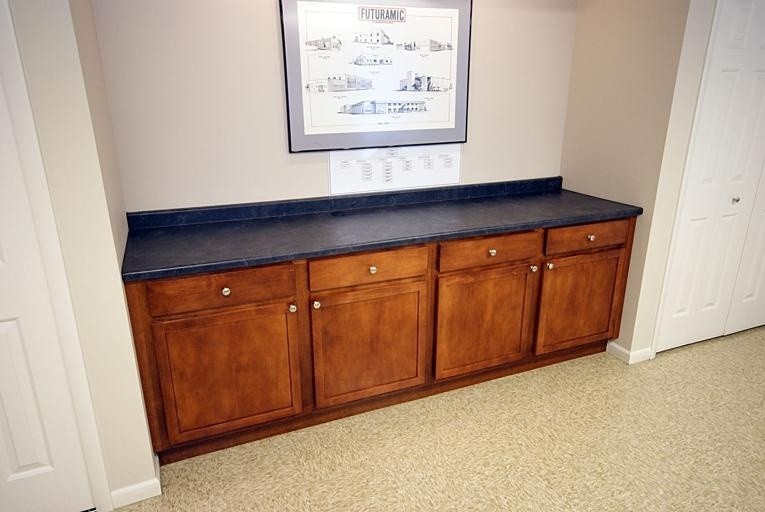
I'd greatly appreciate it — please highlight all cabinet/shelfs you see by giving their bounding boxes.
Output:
[121,197,302,466]
[431,182,534,393]
[532,177,642,366]
[290,190,431,427]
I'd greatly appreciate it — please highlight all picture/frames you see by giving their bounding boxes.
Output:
[277,1,473,152]
